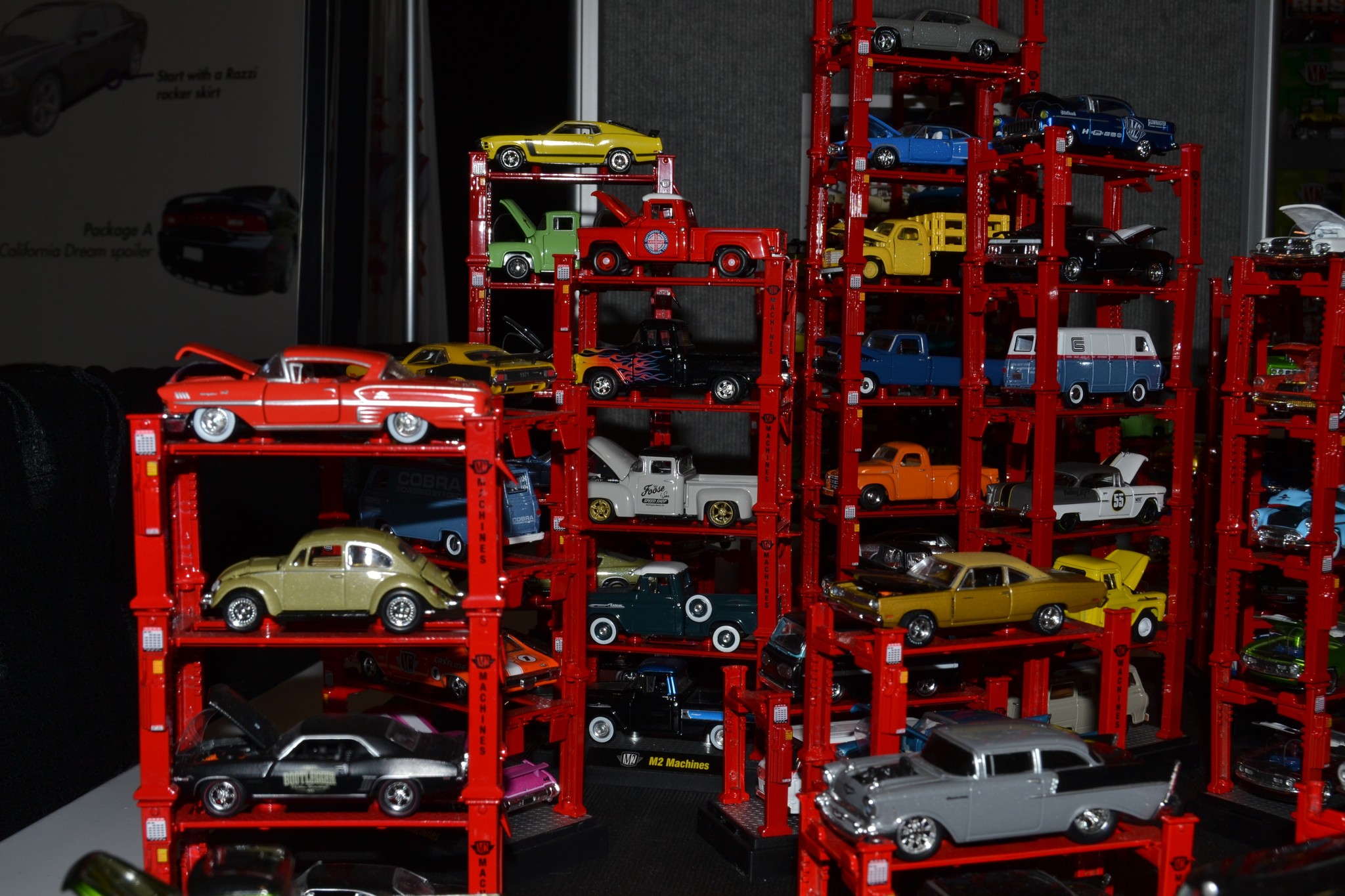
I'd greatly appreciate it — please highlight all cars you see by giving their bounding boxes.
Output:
[4,4,1345,896]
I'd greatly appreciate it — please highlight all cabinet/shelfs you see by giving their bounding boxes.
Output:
[120,4,1345,896]
